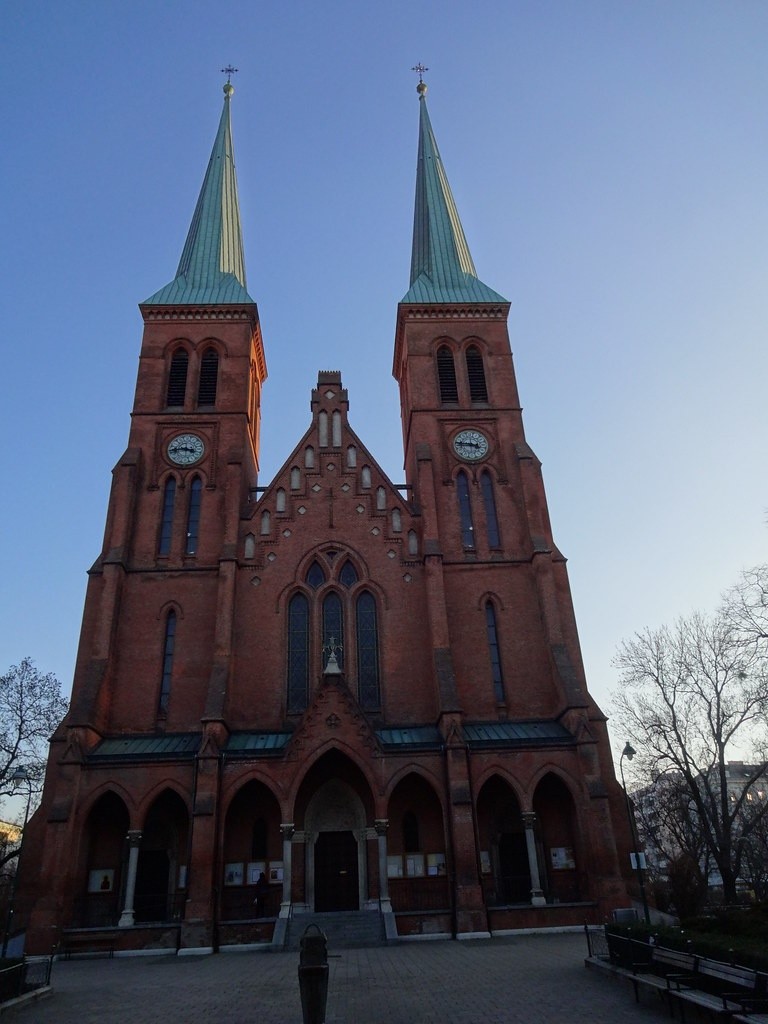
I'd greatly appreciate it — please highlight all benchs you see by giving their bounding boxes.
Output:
[63,932,118,960]
[584,931,768,1024]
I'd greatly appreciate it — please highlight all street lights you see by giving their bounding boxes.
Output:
[619,741,654,942]
[1,765,32,961]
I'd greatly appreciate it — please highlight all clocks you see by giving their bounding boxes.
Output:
[166,433,205,465]
[453,430,489,461]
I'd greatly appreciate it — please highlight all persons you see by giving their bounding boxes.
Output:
[254,871,267,917]
